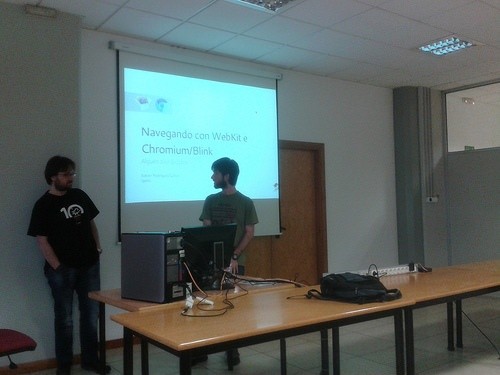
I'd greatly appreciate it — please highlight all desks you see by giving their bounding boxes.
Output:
[110,260,500,375]
[88,276,296,375]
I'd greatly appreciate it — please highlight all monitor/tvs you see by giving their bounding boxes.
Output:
[180,223,237,290]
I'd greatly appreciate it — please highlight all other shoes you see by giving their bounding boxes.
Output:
[225,348,241,366]
[190,355,208,365]
[82,363,111,373]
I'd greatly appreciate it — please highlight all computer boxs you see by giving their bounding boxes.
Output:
[120,233,187,305]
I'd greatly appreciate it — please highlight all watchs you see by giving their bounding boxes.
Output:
[232,254,240,260]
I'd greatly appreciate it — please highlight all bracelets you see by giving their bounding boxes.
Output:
[96,248,102,253]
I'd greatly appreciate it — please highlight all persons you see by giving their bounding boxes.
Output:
[27,156,112,375]
[199,157,258,275]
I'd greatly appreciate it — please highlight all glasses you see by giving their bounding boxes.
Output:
[58,170,77,177]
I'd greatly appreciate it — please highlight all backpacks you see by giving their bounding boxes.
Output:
[305,271,402,305]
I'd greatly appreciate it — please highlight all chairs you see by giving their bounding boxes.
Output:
[0,329,36,369]
